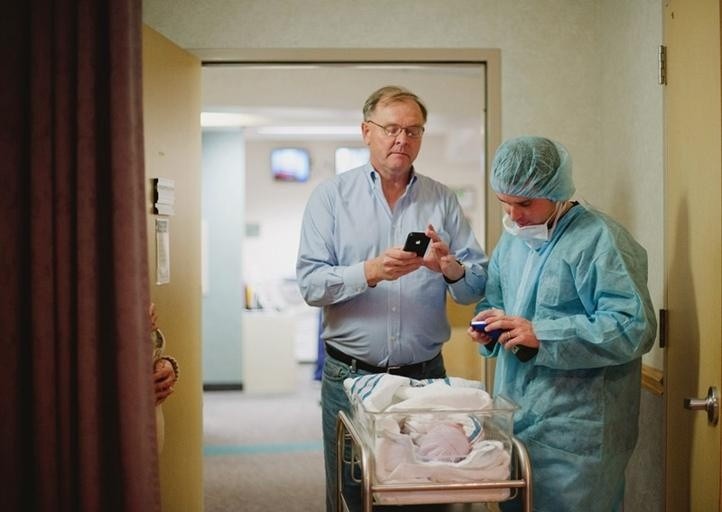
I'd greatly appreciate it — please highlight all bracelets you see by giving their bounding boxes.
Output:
[442,259,467,284]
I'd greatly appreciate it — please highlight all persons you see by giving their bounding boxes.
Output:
[398,409,488,463]
[145,300,181,453]
[290,82,493,511]
[463,132,658,509]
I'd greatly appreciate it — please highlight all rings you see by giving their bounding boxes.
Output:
[507,330,511,339]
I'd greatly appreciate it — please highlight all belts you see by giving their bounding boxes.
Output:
[324,340,442,379]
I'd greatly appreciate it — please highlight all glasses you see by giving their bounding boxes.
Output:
[366,118,426,138]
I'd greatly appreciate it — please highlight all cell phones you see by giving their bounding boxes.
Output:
[470,320,501,338]
[403,231,431,257]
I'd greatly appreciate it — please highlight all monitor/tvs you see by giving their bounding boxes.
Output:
[269,146,311,183]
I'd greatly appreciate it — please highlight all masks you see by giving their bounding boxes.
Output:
[500,212,553,251]
